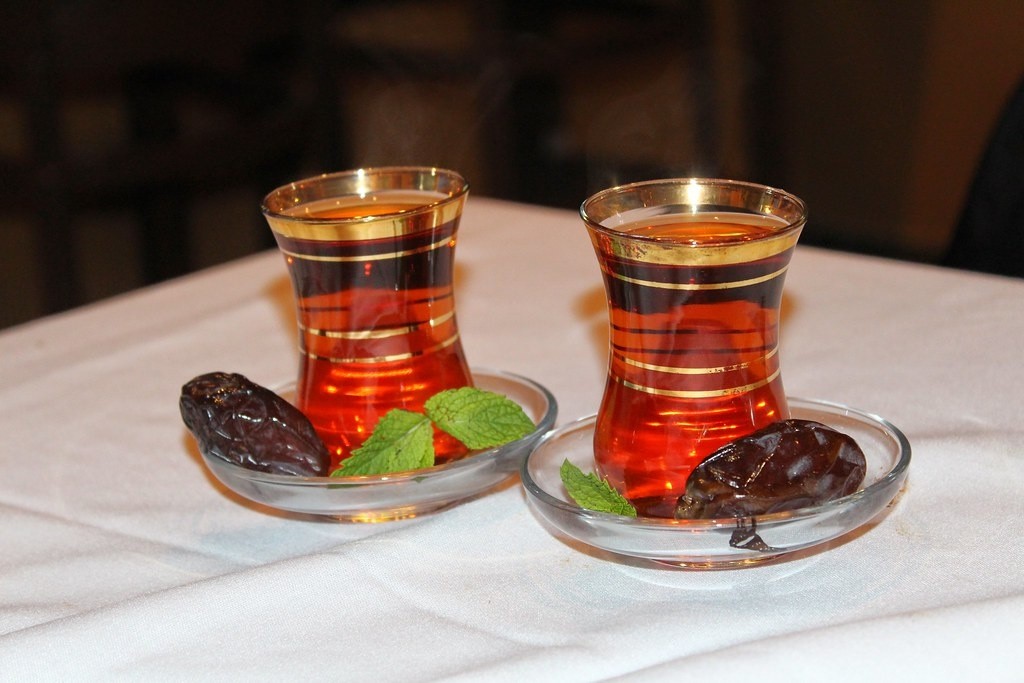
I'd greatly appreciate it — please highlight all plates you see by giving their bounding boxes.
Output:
[523,394,914,572]
[199,365,559,526]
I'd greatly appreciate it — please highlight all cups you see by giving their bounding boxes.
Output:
[263,166,482,479]
[579,181,809,519]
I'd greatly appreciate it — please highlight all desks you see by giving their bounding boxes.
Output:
[1,192,1024,681]
